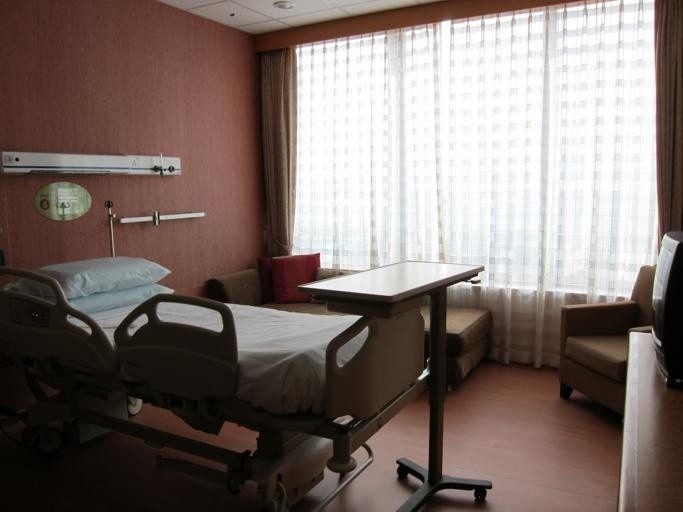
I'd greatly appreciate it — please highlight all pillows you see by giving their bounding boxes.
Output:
[257,256,274,304]
[41,283,175,318]
[272,253,321,304]
[310,267,360,305]
[6,256,172,300]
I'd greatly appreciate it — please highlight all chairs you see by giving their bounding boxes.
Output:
[560,266,657,418]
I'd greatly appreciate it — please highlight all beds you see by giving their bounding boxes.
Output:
[1,264,430,512]
[208,268,493,392]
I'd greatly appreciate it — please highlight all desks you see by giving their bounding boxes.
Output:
[618,331,683,512]
[298,260,492,512]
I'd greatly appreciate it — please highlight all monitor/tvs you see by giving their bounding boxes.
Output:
[649,232,683,390]
